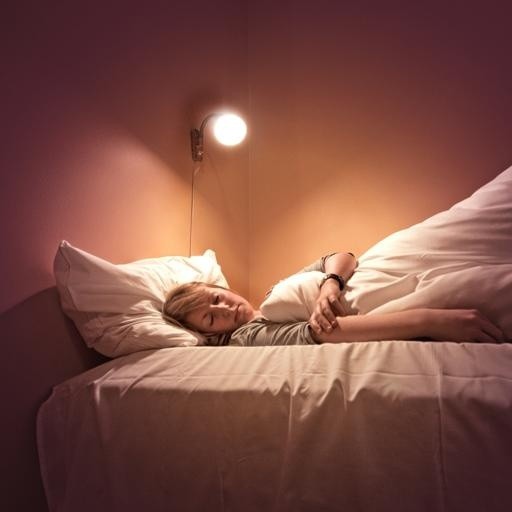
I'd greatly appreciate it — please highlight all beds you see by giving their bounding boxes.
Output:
[36,338,512,512]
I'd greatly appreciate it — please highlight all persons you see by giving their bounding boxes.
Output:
[164,252,503,347]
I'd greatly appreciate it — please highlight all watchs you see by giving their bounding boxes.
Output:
[320,274,343,290]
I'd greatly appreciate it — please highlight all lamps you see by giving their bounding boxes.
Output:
[188,112,248,162]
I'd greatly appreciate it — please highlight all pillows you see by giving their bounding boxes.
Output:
[52,238,232,360]
